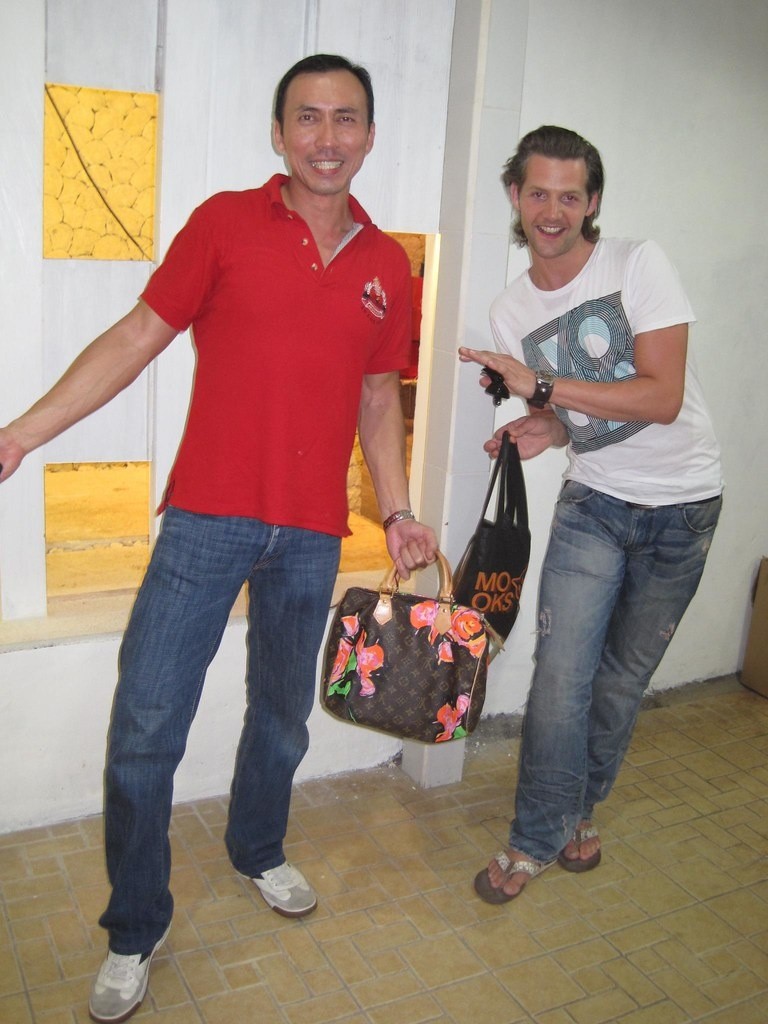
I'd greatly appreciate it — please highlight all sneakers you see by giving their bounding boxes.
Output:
[88,916,174,1024]
[230,861,319,918]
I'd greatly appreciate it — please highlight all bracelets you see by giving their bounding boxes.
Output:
[383,510,414,531]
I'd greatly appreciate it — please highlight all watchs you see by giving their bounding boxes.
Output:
[525,372,555,410]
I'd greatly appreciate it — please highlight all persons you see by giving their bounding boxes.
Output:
[0,53,439,1023]
[459,126,727,906]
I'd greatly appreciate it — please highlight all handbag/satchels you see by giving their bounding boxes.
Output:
[451,430,532,666]
[317,548,491,746]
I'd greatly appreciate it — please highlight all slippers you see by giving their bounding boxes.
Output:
[474,848,558,905]
[558,826,602,874]
